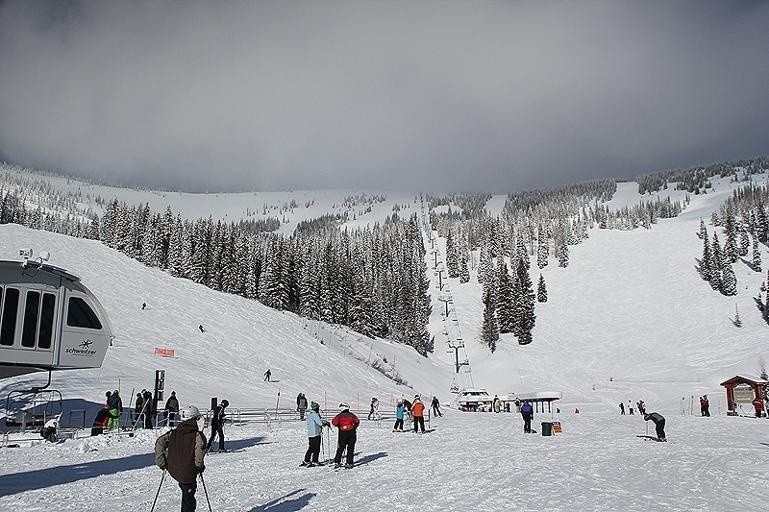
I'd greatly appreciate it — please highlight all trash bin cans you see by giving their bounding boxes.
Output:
[541,422,553,436]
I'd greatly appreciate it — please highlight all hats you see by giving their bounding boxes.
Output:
[338,401,351,410]
[310,401,320,410]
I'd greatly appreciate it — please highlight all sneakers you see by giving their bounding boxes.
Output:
[334,462,354,466]
[303,460,319,465]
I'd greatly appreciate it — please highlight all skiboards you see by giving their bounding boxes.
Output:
[392,429,435,435]
[206,450,246,452]
[299,460,361,469]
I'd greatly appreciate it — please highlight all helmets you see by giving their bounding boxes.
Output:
[181,404,201,423]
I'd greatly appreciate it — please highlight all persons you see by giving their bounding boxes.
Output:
[301,401,331,467]
[618,398,669,441]
[330,402,361,468]
[494,395,501,415]
[700,394,710,417]
[296,392,441,433]
[92,386,231,454]
[142,303,146,310]
[521,400,533,434]
[156,406,213,512]
[199,325,204,333]
[263,368,272,380]
[751,393,769,419]
